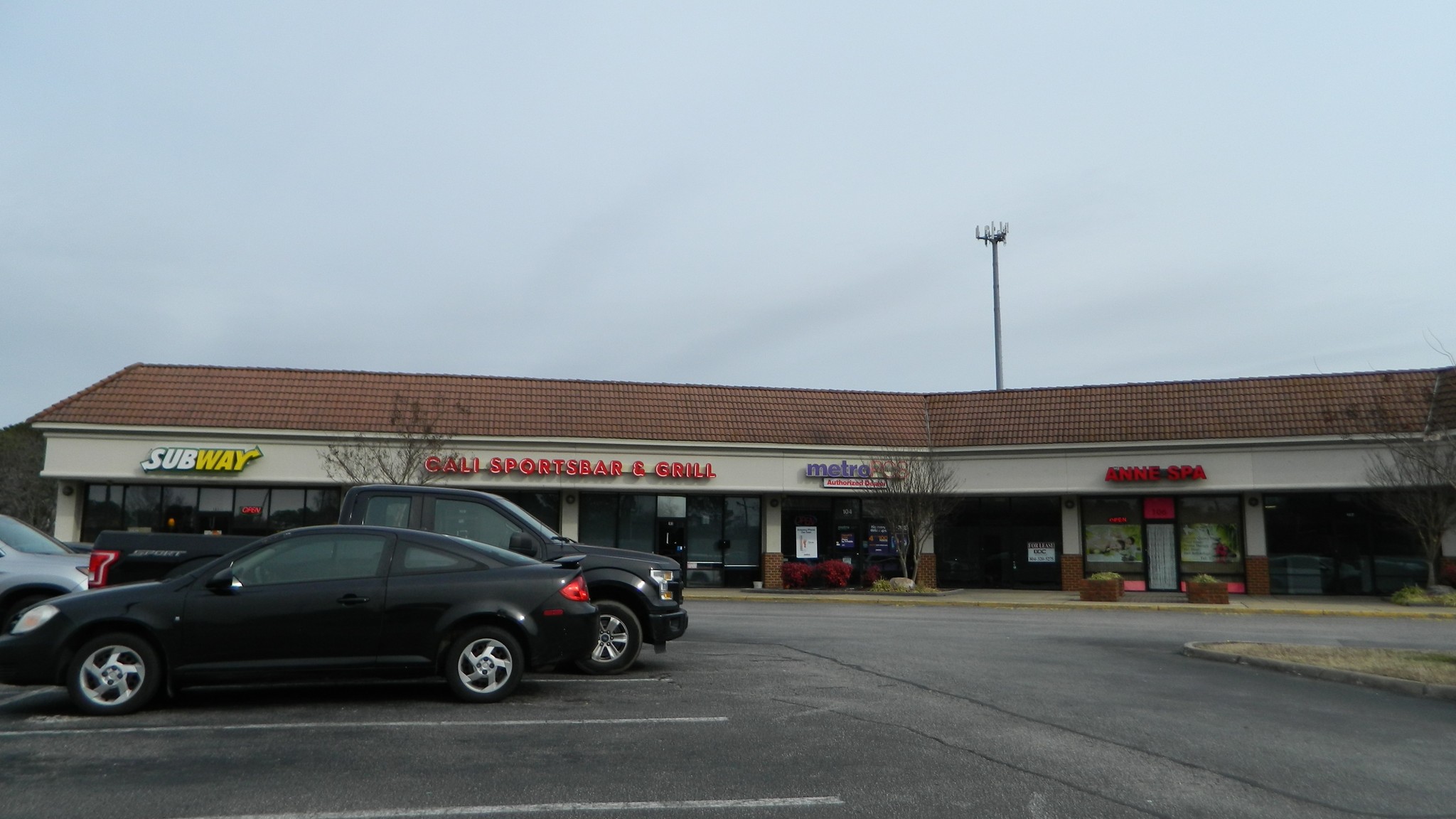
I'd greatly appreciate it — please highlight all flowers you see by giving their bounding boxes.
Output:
[780,561,815,588]
[864,566,878,582]
[815,561,851,587]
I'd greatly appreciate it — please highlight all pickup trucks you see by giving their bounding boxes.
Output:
[89,484,689,676]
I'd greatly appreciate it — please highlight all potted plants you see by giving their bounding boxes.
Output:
[1185,575,1228,604]
[1080,571,1125,601]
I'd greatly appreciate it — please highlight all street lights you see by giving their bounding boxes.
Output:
[975,222,1010,390]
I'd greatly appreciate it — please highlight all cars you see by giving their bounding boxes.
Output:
[0,515,95,633]
[0,525,600,717]
